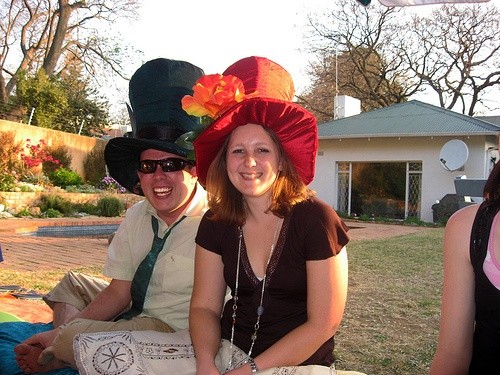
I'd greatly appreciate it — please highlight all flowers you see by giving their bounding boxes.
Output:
[181,73,258,120]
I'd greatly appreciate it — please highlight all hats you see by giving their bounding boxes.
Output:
[193,56,317,195]
[104,59,205,196]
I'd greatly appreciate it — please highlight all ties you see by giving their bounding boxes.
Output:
[113,215,187,322]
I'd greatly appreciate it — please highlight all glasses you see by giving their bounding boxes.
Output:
[138,158,189,174]
